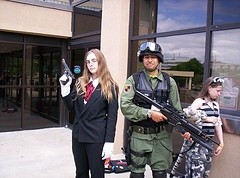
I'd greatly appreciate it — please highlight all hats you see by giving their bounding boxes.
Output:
[138,41,163,63]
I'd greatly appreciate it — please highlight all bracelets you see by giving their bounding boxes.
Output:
[220,145,223,148]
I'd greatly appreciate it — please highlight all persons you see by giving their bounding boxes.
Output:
[119,41,192,178]
[171,77,225,178]
[59,49,118,178]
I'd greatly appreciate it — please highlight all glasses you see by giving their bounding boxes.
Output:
[209,76,223,85]
[139,42,160,52]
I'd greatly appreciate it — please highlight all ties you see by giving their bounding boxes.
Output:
[83,81,94,100]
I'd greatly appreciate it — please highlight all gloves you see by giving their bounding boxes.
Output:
[59,74,72,97]
[101,142,114,160]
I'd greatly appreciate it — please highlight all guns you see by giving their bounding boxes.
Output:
[60,58,75,85]
[135,89,224,155]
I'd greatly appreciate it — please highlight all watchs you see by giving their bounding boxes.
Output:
[147,110,152,118]
